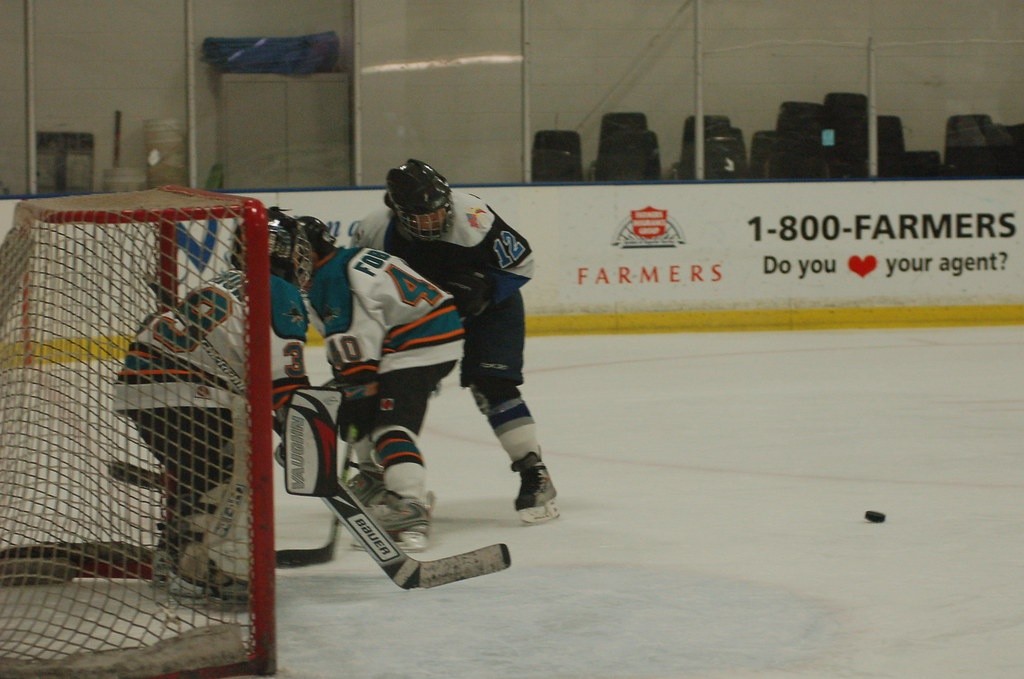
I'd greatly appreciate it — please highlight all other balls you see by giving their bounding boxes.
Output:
[865,511,886,523]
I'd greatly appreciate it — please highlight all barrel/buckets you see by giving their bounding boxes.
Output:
[102,168,148,193]
[144,119,187,189]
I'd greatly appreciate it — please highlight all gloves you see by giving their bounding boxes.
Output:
[339,400,381,444]
[441,277,477,315]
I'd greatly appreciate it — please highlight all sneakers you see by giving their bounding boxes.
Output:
[351,490,436,553]
[152,551,251,604]
[346,469,387,508]
[510,453,559,523]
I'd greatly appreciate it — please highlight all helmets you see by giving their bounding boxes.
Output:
[232,206,302,278]
[295,216,336,259]
[387,160,453,215]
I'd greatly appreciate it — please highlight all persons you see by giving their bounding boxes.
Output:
[111,209,312,606]
[349,158,560,523]
[297,216,464,552]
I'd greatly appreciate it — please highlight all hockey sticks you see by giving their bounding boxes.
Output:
[140,268,514,593]
[275,426,357,571]
[106,376,336,490]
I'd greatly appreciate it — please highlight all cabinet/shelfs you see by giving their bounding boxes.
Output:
[211,73,350,192]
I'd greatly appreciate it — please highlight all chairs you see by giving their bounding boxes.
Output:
[532,92,1024,181]
